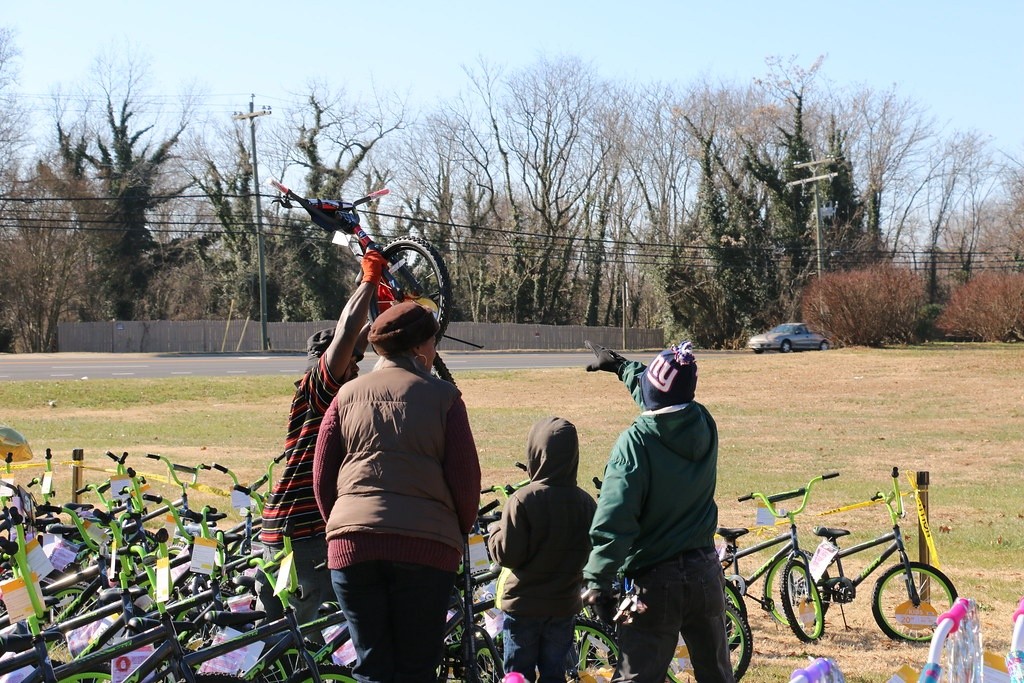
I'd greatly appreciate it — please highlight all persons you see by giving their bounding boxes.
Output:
[488,417,597,683]
[315,302,482,683]
[582,339,734,683]
[254,252,387,643]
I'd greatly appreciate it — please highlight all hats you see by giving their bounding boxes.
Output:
[304,327,365,375]
[367,301,440,360]
[638,340,700,412]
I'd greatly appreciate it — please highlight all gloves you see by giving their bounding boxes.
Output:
[582,337,629,382]
[360,249,390,286]
[587,588,618,627]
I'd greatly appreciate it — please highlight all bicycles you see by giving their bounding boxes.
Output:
[267,178,486,397]
[710,472,844,656]
[764,467,961,643]
[1,426,756,683]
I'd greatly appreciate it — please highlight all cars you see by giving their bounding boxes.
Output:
[748,322,834,353]
